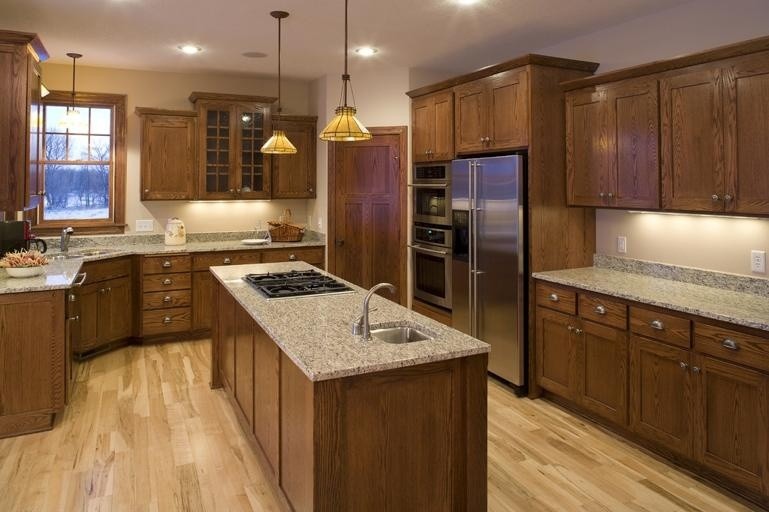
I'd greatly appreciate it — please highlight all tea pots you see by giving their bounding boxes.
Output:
[165,217,186,245]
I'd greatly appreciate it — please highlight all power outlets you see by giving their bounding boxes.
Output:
[751,250,766,274]
[618,237,627,253]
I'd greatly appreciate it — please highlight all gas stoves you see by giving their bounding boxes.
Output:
[240,268,355,300]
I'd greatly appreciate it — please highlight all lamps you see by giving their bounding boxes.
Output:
[318,0,373,142]
[62,53,85,129]
[261,11,298,155]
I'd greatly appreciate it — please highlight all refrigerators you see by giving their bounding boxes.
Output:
[451,149,528,395]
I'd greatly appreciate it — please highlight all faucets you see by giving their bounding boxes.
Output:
[60,227,74,252]
[360,280,396,338]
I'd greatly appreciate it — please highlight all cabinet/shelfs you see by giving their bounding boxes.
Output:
[59,246,136,359]
[270,113,318,201]
[279,326,491,512]
[405,76,454,329]
[257,241,326,275]
[629,274,769,501]
[0,29,50,209]
[531,265,629,432]
[559,58,661,212]
[137,243,192,340]
[658,35,769,215]
[253,306,448,476]
[235,288,391,434]
[0,262,86,439]
[208,266,352,404]
[191,240,261,338]
[135,106,199,200]
[449,54,596,401]
[188,91,278,199]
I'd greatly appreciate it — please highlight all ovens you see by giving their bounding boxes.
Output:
[406,224,452,315]
[406,161,452,231]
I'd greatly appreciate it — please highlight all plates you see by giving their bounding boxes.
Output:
[3,263,48,278]
[239,238,268,244]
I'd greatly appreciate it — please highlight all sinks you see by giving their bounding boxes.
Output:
[52,248,117,257]
[369,320,436,346]
